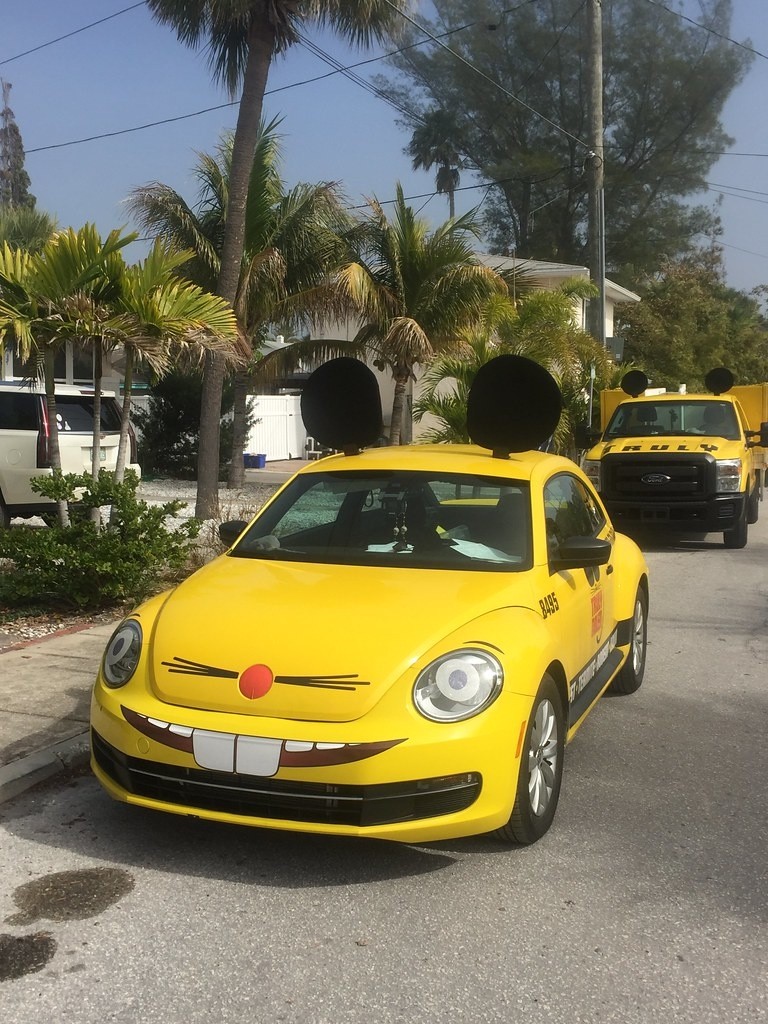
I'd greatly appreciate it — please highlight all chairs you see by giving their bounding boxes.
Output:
[699,405,729,433]
[346,509,395,547]
[486,491,526,552]
[630,405,665,435]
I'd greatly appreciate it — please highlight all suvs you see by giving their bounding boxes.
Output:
[0,380,144,530]
[582,366,768,546]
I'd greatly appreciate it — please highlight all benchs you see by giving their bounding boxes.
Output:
[424,506,499,540]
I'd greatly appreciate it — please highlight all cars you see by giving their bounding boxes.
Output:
[87,356,653,849]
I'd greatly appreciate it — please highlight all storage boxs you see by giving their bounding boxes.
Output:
[243,453,267,468]
